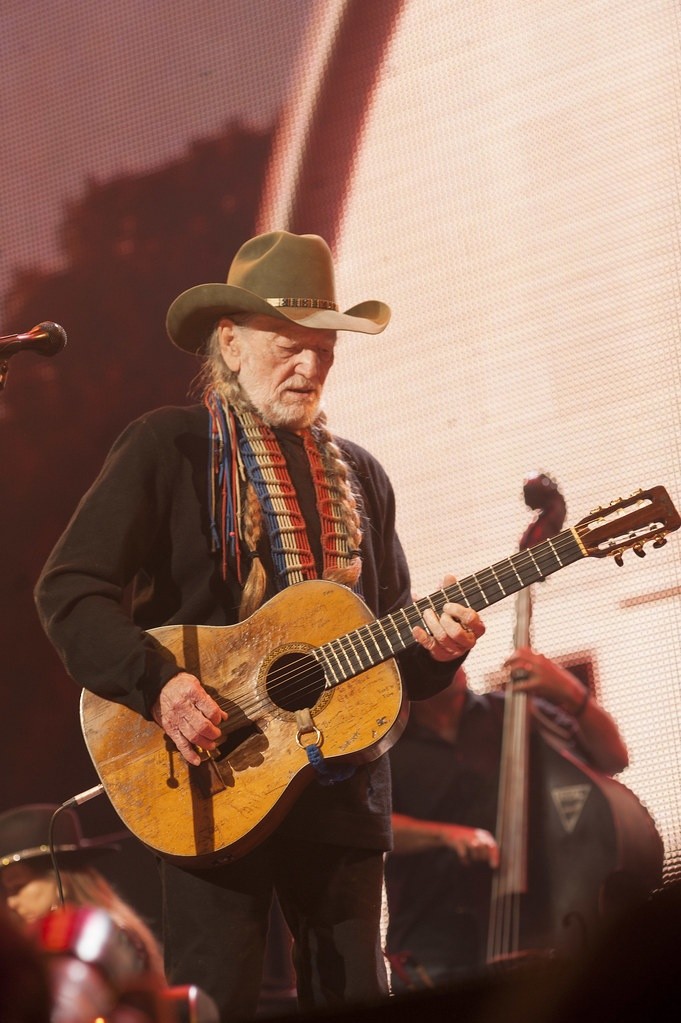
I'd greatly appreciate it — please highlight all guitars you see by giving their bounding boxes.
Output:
[80,485,681,869]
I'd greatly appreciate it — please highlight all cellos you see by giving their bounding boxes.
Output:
[406,475,666,1023]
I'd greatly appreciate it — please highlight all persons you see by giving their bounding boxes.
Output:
[33,230,484,1022]
[383,644,630,927]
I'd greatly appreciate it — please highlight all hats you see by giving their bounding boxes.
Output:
[0,803,120,907]
[166,231,389,355]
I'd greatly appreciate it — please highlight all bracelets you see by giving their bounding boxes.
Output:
[576,686,590,715]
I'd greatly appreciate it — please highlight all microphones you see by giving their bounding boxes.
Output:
[0,321,68,358]
